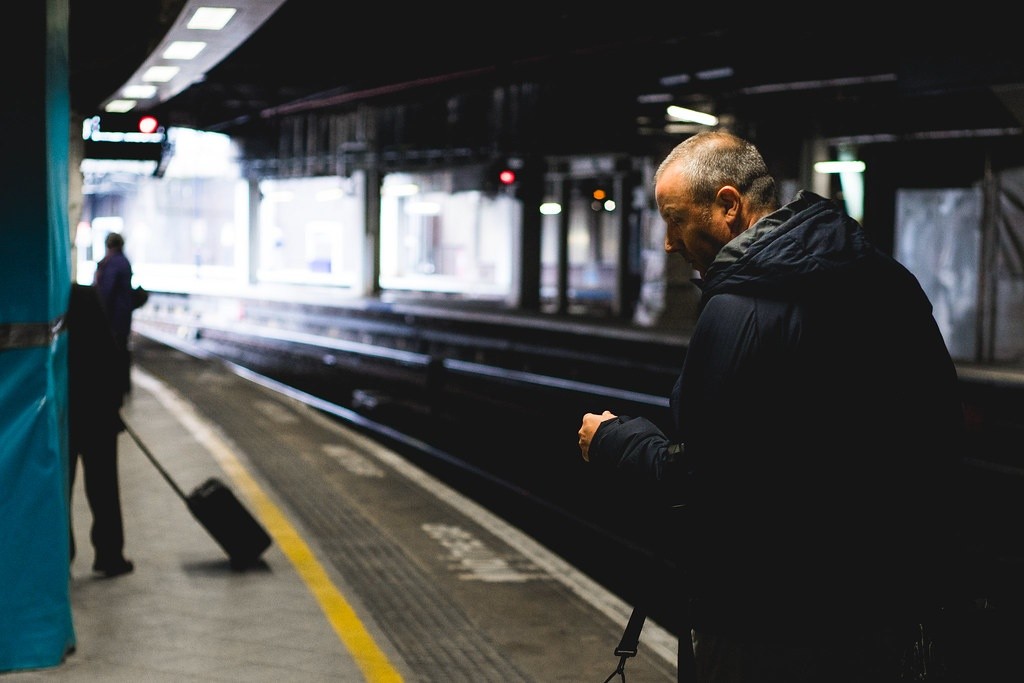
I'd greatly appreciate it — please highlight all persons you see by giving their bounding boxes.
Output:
[64,275,134,574]
[92,232,133,402]
[577,130,968,683]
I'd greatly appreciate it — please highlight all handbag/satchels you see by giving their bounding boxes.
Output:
[118,286,149,312]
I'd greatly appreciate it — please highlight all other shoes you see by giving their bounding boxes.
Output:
[92,556,134,577]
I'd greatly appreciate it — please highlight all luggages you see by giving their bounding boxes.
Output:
[125,425,273,571]
